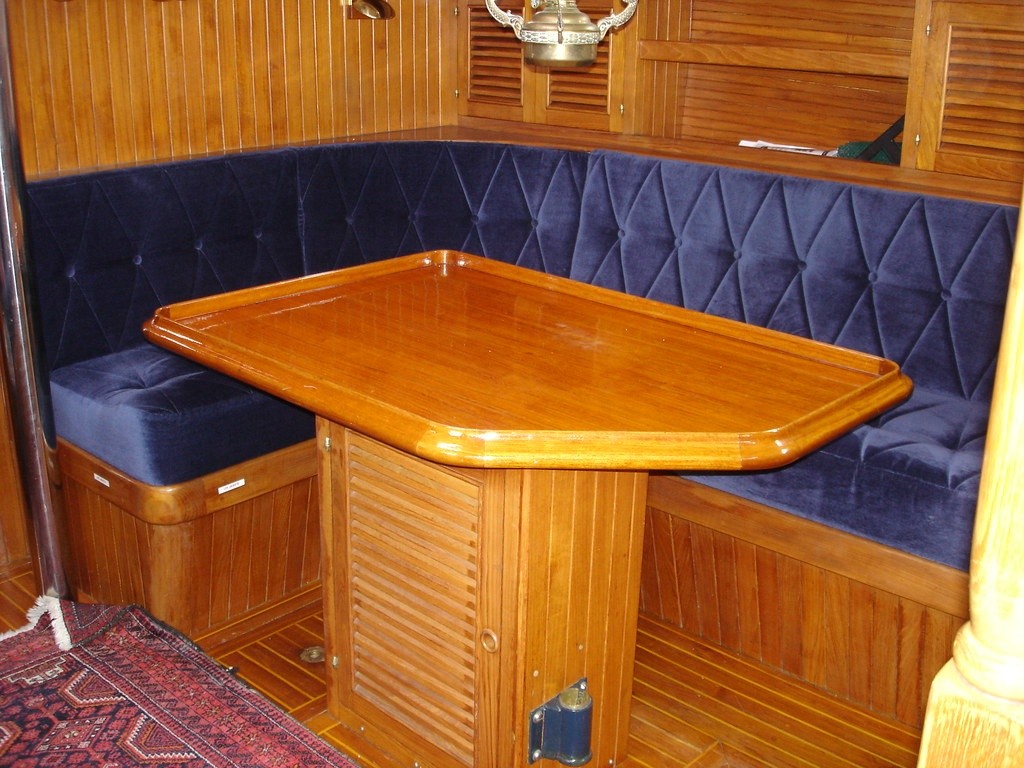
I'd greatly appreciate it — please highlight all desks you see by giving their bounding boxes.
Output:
[139,249,912,766]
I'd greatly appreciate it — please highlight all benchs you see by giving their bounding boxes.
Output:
[25,137,1020,731]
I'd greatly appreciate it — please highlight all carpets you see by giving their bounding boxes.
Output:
[1,592,356,767]
